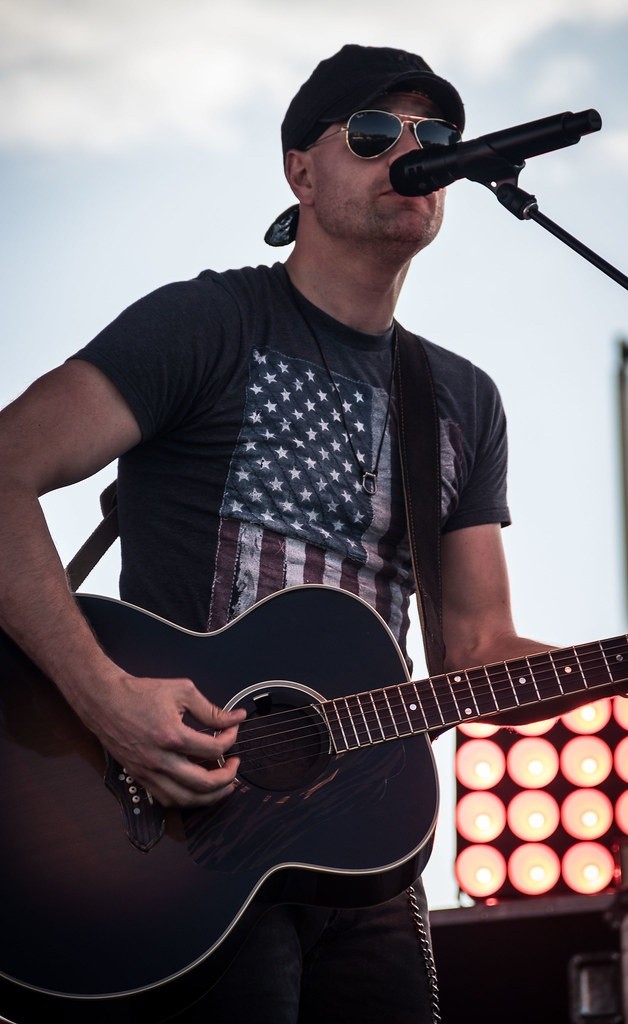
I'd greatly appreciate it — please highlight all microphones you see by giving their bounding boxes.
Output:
[389,109,602,196]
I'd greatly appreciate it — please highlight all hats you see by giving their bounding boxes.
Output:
[264,44,465,247]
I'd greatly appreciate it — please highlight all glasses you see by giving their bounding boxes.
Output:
[303,110,462,159]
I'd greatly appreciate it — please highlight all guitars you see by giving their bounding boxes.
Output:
[0,582,628,1024]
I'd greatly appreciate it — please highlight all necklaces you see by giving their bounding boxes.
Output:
[277,263,400,497]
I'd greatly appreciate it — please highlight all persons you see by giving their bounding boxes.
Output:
[0,41,568,1023]
